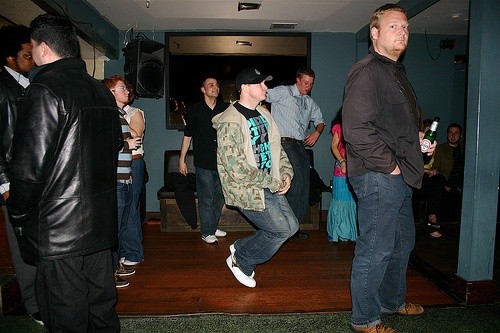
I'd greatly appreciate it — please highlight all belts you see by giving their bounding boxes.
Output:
[281,137,302,143]
[132,155,142,158]
[117,179,131,184]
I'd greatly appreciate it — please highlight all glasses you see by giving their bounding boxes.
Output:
[448,132,459,135]
[111,87,127,90]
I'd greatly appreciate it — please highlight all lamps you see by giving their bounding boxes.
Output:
[440,39,458,50]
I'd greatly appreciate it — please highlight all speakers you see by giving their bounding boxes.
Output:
[123,37,166,99]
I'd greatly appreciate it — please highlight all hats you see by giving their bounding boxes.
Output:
[236,68,273,90]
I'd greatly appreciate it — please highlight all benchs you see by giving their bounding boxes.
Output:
[157,150,322,233]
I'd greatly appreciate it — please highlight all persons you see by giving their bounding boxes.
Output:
[96,74,141,288]
[431,123,466,224]
[210,66,299,289]
[179,77,232,242]
[115,81,148,266]
[415,118,447,240]
[328,104,361,247]
[345,3,425,333]
[0,21,67,328]
[257,68,326,241]
[7,11,126,333]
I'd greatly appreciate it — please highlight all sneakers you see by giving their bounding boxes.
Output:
[113,267,135,276]
[382,303,424,316]
[230,244,254,278]
[113,274,129,287]
[214,229,226,236]
[226,255,256,288]
[201,234,218,244]
[350,323,399,333]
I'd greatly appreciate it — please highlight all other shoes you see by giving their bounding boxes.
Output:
[124,259,144,266]
[328,235,338,243]
[428,214,437,224]
[30,312,44,326]
[340,236,349,242]
[294,229,310,240]
[431,231,442,238]
[119,257,125,263]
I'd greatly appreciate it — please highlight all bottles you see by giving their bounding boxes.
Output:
[420,117,440,165]
